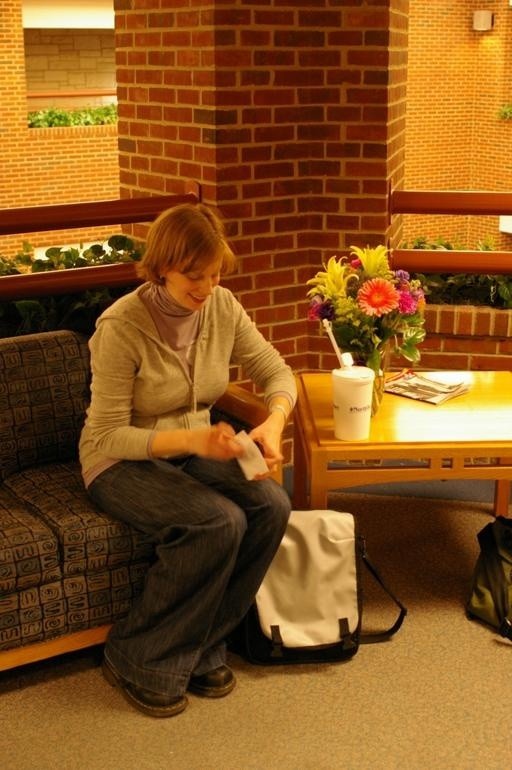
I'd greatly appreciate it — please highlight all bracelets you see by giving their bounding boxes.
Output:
[268,406,288,423]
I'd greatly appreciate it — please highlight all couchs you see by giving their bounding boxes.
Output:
[0,325,287,683]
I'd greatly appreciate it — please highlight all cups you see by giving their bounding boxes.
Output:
[330,366,375,444]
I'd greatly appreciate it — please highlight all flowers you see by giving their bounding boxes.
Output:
[304,244,427,373]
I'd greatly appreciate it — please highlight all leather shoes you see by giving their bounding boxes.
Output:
[185,665,236,699]
[101,657,190,720]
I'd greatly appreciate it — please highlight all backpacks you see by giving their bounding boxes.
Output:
[243,506,407,667]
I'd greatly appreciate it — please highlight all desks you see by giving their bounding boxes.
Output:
[292,368,512,520]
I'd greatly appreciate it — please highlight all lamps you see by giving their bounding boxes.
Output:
[471,9,494,32]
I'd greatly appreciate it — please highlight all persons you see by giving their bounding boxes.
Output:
[72,198,300,720]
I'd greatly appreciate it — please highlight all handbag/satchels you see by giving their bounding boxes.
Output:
[464,515,512,642]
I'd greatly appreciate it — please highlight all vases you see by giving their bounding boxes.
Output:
[371,364,386,416]
